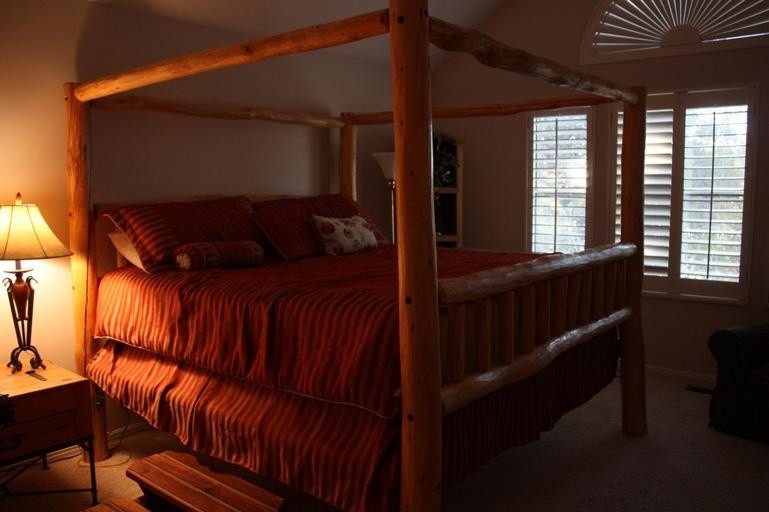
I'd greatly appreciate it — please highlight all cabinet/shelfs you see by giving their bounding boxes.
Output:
[433,142,465,249]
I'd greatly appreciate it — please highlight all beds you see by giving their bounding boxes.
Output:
[65,192,647,512]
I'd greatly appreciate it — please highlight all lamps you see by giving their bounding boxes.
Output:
[373,152,396,246]
[0,191,76,375]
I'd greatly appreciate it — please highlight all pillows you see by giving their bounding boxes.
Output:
[103,193,391,276]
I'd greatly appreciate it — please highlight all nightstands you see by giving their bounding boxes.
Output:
[0,355,100,507]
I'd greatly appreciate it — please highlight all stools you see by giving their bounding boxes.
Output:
[707,324,767,442]
